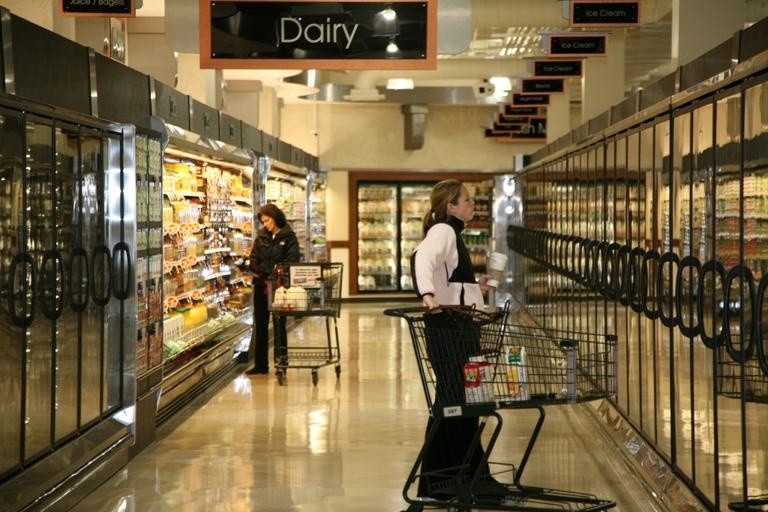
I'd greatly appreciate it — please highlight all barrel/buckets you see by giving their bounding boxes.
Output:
[272,285,310,311]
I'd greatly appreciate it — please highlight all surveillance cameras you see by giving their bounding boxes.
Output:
[474,83,495,97]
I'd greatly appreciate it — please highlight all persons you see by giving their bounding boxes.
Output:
[409,178,510,505]
[235,203,299,376]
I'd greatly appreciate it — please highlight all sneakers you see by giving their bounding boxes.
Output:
[275,365,287,375]
[246,363,270,377]
[416,476,511,508]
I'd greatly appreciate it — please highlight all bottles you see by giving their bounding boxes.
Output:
[463,354,495,404]
[461,183,489,272]
[285,286,309,309]
[503,344,532,403]
[163,158,253,359]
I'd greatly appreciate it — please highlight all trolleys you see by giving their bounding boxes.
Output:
[268,258,352,387]
[376,304,632,511]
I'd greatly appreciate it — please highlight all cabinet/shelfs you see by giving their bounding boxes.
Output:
[265,175,307,269]
[162,141,258,420]
[656,171,768,332]
[307,183,328,268]
